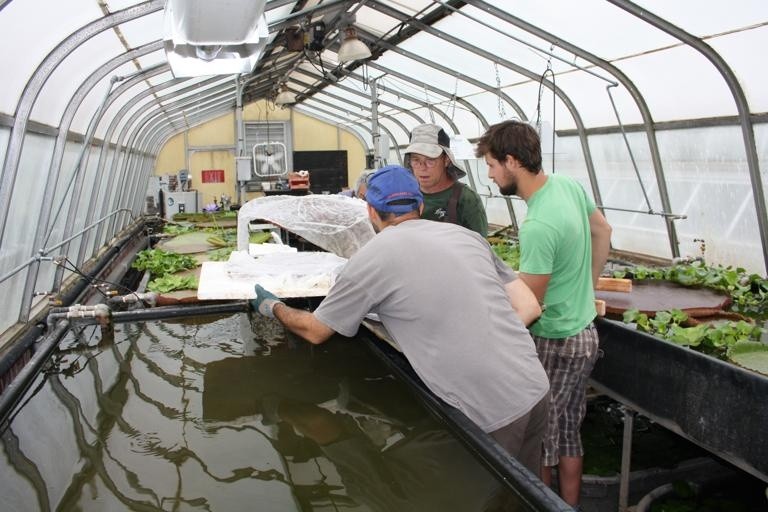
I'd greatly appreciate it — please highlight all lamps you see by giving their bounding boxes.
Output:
[337,23,371,60]
[273,83,297,104]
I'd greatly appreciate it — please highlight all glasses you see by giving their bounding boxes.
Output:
[365,173,383,195]
[407,158,435,168]
[408,155,446,168]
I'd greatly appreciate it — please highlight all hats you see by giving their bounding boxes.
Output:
[403,123,465,180]
[364,165,423,216]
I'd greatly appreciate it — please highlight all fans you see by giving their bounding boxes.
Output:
[253,142,287,177]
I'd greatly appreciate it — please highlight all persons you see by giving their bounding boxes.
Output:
[473,120,612,510]
[251,166,550,481]
[401,124,488,237]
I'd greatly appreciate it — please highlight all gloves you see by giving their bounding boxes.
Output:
[251,284,285,319]
